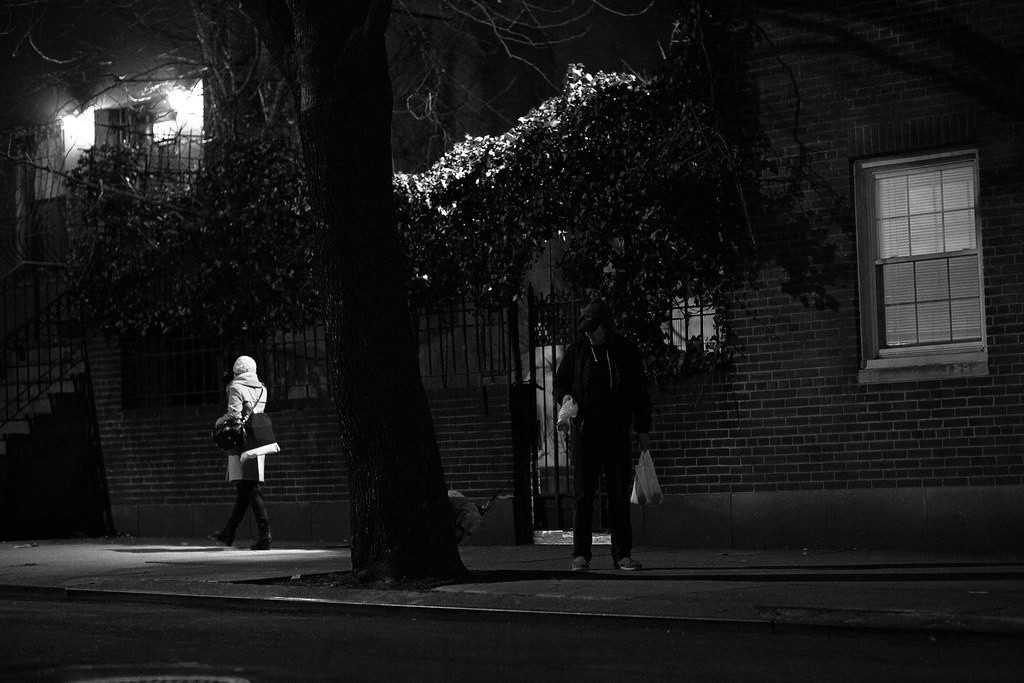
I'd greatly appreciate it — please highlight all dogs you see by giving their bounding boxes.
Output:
[448,490,485,546]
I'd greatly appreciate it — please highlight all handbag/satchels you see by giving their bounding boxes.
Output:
[211,416,248,453]
[630,450,664,507]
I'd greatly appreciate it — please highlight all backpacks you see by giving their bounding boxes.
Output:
[239,409,280,463]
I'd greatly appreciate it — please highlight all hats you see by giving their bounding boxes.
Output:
[576,316,601,333]
[232,355,257,373]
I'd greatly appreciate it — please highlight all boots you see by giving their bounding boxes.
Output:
[212,519,239,546]
[250,523,273,550]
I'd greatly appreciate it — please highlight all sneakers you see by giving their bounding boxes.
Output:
[614,556,643,570]
[571,555,590,570]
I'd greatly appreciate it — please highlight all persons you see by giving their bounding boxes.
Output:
[551,299,653,572]
[209,356,273,551]
